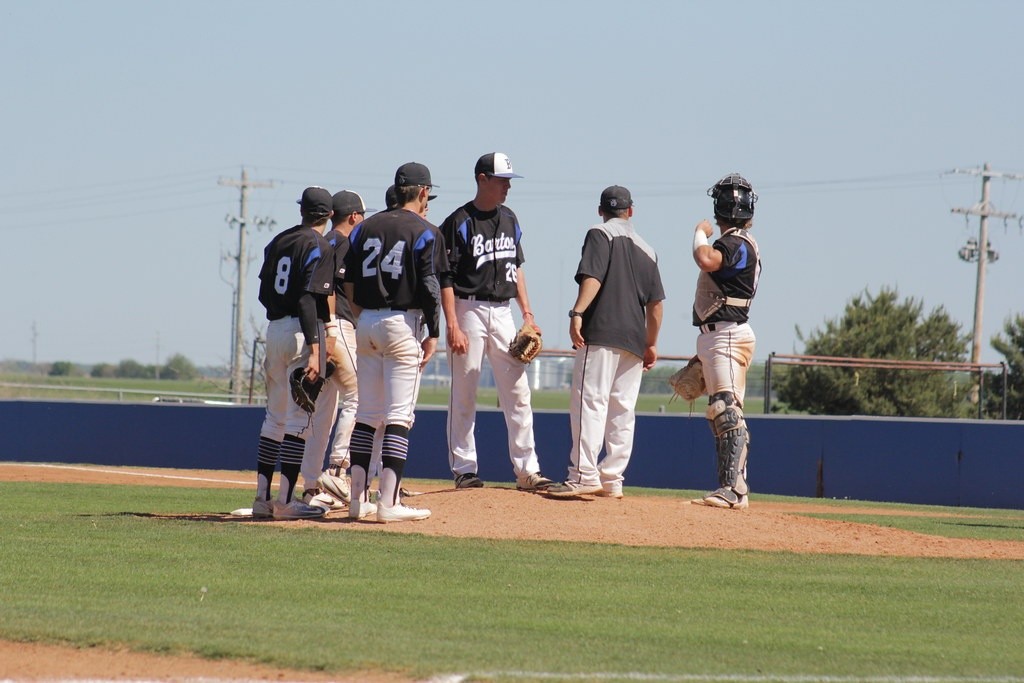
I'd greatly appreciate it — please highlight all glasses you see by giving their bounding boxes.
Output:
[417,184,434,192]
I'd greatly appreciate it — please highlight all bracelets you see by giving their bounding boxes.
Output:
[522,312,534,318]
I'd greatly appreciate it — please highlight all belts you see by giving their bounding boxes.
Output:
[391,307,407,312]
[699,322,744,334]
[291,314,299,318]
[459,295,509,304]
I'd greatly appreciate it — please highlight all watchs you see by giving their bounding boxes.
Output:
[569,310,584,317]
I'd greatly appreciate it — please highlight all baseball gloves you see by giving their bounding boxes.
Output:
[288,366,326,415]
[509,324,542,366]
[667,354,707,402]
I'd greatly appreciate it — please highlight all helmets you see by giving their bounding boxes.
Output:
[707,172,758,219]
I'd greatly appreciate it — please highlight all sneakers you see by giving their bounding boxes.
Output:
[600,491,623,498]
[349,500,377,518]
[547,479,603,496]
[303,492,345,509]
[455,472,483,488]
[691,499,705,506]
[252,496,274,518]
[317,469,349,502]
[516,471,556,489]
[272,496,325,519]
[705,495,749,509]
[377,502,431,523]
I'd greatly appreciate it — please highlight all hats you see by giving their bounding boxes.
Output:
[333,190,377,214]
[385,184,438,206]
[297,187,333,215]
[601,185,633,208]
[475,152,525,178]
[395,162,439,188]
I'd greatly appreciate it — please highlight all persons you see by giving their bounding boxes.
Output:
[691,173,761,510]
[439,151,554,490]
[253,162,451,523]
[547,185,666,498]
